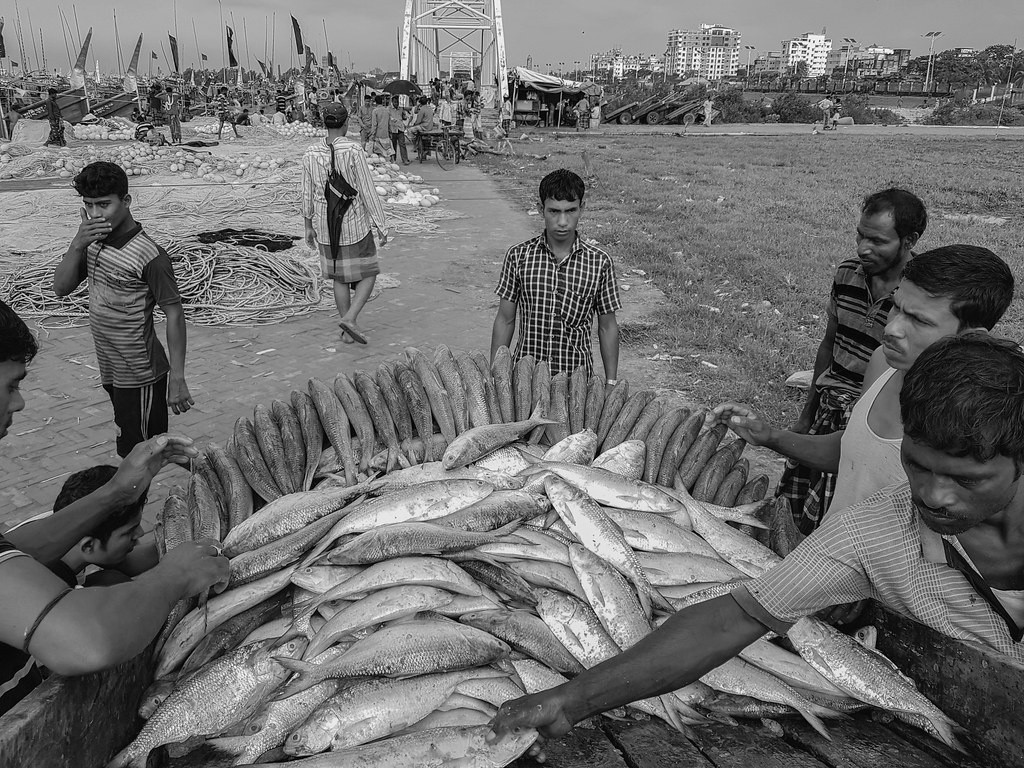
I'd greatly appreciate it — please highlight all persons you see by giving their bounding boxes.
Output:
[43,75,600,166]
[700,95,951,130]
[302,101,389,345]
[770,187,929,535]
[52,161,194,463]
[0,298,230,721]
[703,245,1015,624]
[489,168,622,399]
[6,464,161,590]
[488,334,1024,764]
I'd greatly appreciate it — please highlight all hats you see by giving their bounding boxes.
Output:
[503,94,508,97]
[584,94,589,97]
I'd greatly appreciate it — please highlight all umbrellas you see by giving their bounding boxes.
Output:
[382,80,422,95]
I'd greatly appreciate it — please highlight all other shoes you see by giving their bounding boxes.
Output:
[406,160,411,164]
[341,332,354,343]
[339,317,368,344]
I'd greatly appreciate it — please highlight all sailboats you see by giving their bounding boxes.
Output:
[0,0,349,139]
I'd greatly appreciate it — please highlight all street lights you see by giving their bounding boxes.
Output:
[839,38,862,88]
[919,32,947,92]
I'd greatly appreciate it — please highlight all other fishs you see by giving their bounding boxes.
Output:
[104,398,969,768]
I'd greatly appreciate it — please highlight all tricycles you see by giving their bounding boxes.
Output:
[413,122,465,171]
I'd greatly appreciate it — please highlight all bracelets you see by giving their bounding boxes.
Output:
[606,379,618,386]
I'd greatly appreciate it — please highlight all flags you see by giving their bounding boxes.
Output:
[11,15,335,75]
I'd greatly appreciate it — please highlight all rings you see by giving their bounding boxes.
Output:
[384,241,386,243]
[210,543,223,558]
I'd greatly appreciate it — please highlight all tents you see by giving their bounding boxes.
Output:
[507,64,603,128]
